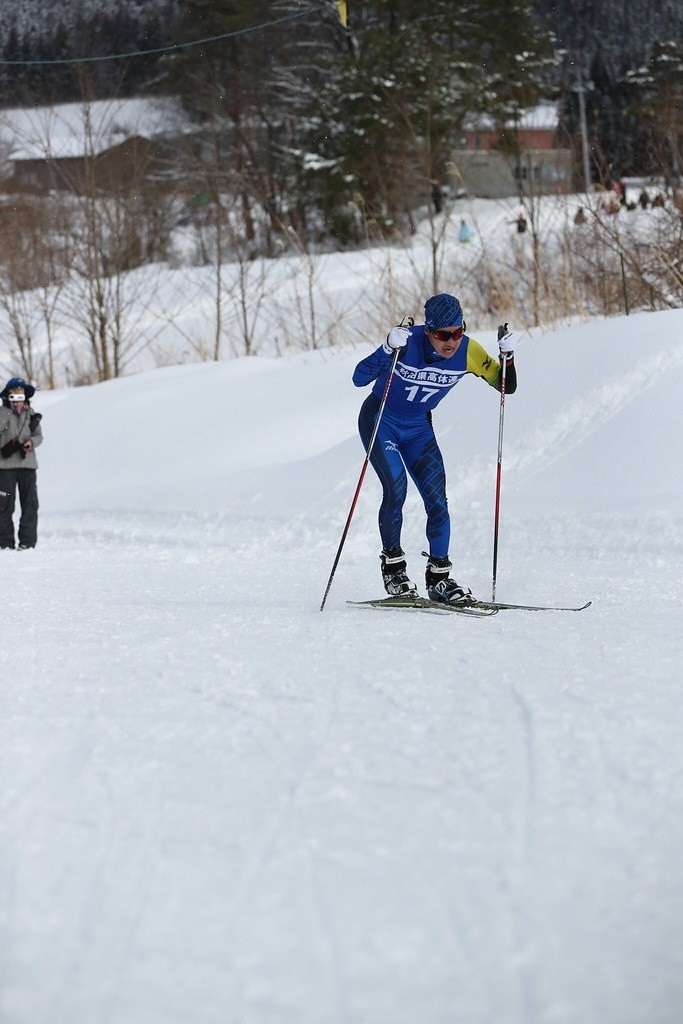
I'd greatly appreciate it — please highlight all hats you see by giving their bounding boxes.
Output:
[425,293,463,329]
[0,377,35,400]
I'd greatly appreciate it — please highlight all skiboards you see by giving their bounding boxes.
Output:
[348,600,594,616]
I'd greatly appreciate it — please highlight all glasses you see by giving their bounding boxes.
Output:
[427,321,466,341]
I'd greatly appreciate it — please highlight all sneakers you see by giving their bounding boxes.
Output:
[380,549,417,594]
[421,551,464,602]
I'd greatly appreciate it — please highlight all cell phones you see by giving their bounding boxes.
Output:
[22,443,30,450]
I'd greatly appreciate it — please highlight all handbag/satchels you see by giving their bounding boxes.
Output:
[2,437,22,457]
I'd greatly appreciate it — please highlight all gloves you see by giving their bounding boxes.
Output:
[383,327,413,354]
[497,332,515,352]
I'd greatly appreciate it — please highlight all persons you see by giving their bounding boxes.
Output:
[0,378,43,551]
[574,173,664,224]
[352,293,517,604]
[515,214,527,233]
[458,220,471,243]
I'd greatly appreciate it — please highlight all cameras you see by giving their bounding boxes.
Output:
[8,394,25,401]
